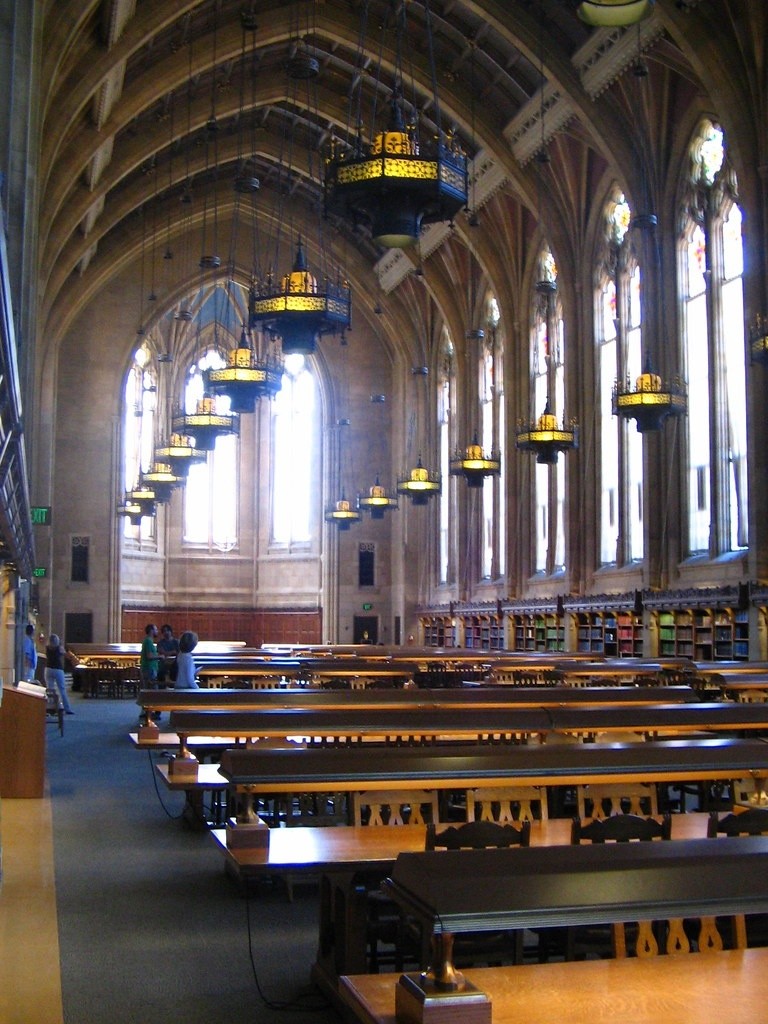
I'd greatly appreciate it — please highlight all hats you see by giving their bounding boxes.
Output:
[179,632,198,653]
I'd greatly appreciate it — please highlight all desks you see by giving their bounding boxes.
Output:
[129,732,586,822]
[153,762,230,824]
[209,808,768,992]
[335,947,768,1024]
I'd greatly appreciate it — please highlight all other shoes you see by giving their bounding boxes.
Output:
[154,714,161,721]
[65,710,74,714]
[139,712,156,720]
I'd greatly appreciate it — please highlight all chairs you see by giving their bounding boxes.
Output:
[91,660,768,1024]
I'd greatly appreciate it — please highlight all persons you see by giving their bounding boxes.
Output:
[173,631,200,689]
[44,633,77,715]
[137,623,166,720]
[154,624,180,720]
[23,624,38,682]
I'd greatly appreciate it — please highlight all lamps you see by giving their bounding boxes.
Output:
[451,616,456,628]
[116,0,689,527]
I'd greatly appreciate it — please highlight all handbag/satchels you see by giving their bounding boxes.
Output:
[168,660,178,681]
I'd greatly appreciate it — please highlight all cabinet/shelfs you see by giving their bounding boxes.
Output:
[515,613,566,653]
[653,609,749,661]
[423,618,456,647]
[465,615,503,651]
[576,611,644,658]
